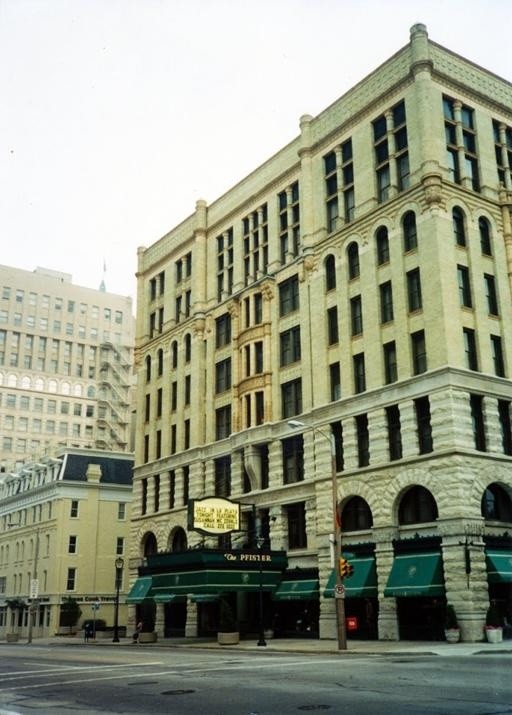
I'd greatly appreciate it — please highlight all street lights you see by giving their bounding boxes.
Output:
[112,557,125,645]
[286,419,347,654]
